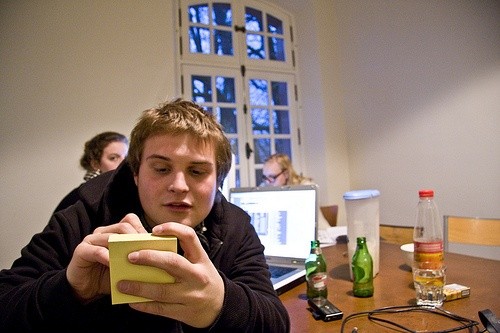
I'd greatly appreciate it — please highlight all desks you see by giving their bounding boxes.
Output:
[275,238,500,333]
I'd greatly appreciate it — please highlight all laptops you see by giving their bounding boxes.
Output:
[228,183,320,296]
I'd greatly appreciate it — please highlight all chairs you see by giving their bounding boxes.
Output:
[442,214,500,253]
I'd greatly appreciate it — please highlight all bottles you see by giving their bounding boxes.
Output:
[304,240,328,300]
[413,189,445,264]
[351,237,374,299]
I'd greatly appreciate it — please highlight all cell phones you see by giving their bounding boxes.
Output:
[307,296,343,322]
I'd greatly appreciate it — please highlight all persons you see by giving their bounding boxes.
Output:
[0,99,291,333]
[80,132,130,182]
[260,154,330,231]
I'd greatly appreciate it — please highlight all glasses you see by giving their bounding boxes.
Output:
[262,168,287,183]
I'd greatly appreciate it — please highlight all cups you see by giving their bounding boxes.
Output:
[412,261,447,309]
[342,189,380,280]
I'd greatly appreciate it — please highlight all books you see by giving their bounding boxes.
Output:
[317,225,350,249]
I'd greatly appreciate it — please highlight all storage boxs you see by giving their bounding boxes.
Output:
[428,283,471,302]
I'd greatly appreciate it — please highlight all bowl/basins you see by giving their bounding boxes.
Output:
[399,243,414,268]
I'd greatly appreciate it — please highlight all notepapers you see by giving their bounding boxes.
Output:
[108,235,177,305]
